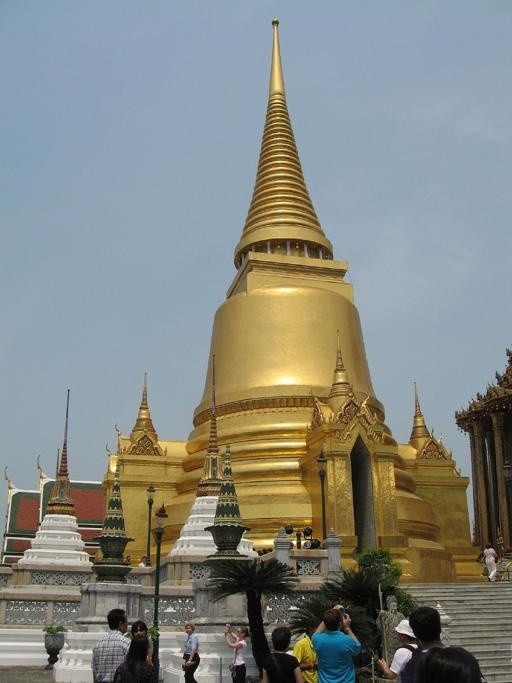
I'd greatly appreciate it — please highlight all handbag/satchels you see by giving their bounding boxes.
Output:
[483,567,488,576]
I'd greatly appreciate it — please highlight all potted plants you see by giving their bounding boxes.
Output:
[43,624,68,670]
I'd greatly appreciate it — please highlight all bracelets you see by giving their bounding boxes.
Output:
[189,656,193,660]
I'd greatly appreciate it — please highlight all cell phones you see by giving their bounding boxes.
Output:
[339,608,346,621]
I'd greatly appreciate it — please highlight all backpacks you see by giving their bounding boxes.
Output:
[396,643,422,659]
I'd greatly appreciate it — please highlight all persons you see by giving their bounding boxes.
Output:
[91,609,132,683]
[259,603,481,681]
[483,542,499,581]
[225,622,250,682]
[131,618,154,664]
[182,622,201,682]
[113,635,159,683]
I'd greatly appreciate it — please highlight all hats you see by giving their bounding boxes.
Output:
[395,620,416,638]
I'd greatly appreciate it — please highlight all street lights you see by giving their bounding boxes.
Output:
[317,450,327,549]
[285,524,312,575]
[150,503,168,682]
[146,483,155,567]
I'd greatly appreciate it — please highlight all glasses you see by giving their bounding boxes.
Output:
[133,629,145,632]
